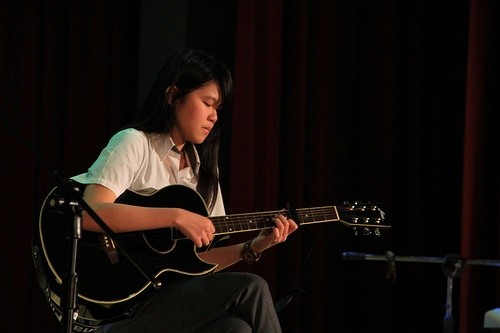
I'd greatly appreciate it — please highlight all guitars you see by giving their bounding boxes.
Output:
[34,179,392,328]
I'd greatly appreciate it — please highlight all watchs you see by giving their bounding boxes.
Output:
[242,239,262,265]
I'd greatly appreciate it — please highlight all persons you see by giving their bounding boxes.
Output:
[69,47,299,333]
